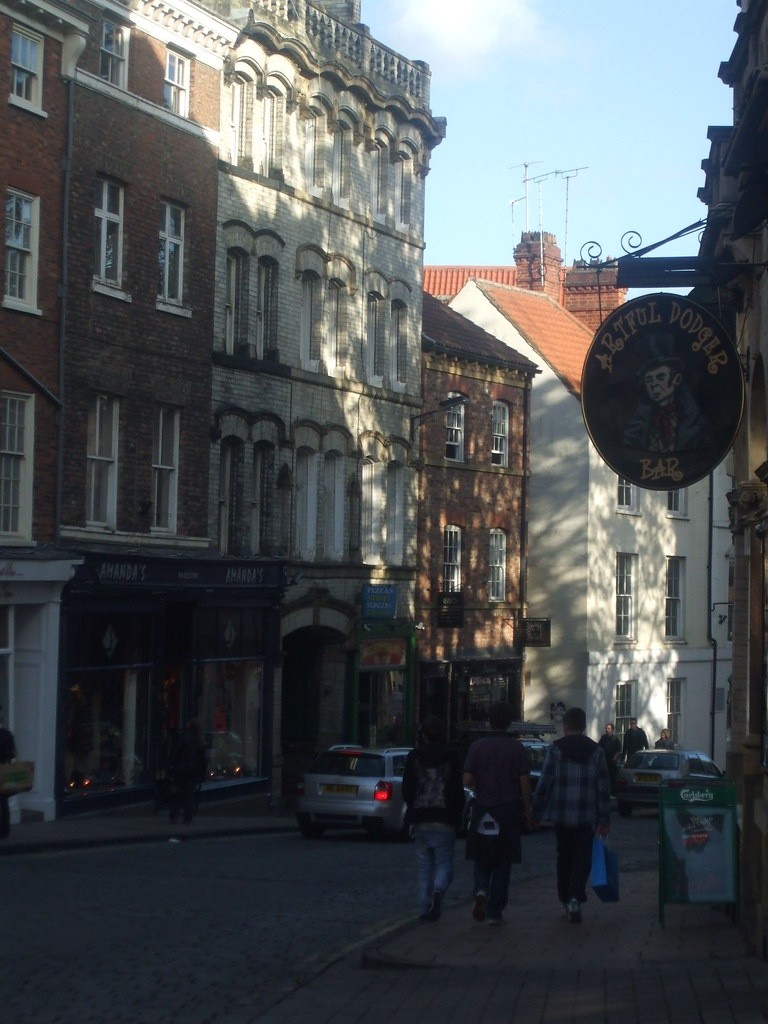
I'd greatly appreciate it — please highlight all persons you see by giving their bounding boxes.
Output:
[401,713,461,923]
[0,715,17,838]
[461,701,532,925]
[599,717,676,796]
[167,718,200,824]
[530,708,612,923]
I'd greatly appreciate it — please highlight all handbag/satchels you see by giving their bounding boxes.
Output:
[0,750,35,794]
[590,828,621,904]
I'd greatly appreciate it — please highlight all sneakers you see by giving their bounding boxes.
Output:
[568,897,583,923]
[472,890,488,923]
[575,876,588,904]
[419,913,427,923]
[487,910,506,925]
[428,889,443,921]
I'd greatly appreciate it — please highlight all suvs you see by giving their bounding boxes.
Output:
[294,744,476,843]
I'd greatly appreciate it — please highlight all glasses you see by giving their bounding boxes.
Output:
[631,723,636,724]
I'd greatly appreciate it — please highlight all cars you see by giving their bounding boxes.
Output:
[613,748,728,817]
[520,741,552,795]
